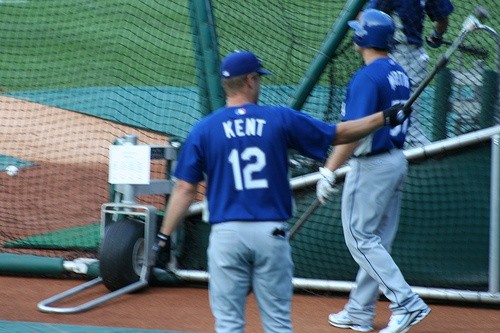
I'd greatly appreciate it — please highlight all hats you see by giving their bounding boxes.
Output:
[220,51,271,78]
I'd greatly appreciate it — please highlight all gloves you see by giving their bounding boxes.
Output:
[383,104,412,126]
[316,165,340,206]
[425,28,442,48]
[152,232,170,268]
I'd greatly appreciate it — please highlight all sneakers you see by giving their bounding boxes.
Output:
[380,302,431,333]
[327,309,371,333]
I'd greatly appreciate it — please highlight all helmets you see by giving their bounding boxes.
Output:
[346,9,394,49]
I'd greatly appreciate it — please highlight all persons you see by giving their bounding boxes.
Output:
[152,50,413,333]
[316,9,431,333]
[357,0,454,150]
